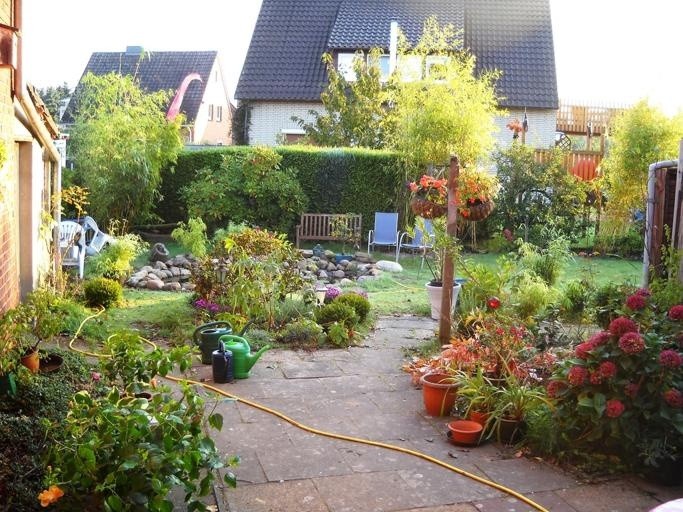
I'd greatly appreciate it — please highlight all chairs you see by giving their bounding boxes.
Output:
[51,220,84,278]
[77,216,117,256]
[368,212,401,260]
[397,218,440,268]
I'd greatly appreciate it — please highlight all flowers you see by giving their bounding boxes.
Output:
[452,171,496,219]
[461,318,537,376]
[410,175,449,205]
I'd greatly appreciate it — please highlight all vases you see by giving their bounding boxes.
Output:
[411,195,446,218]
[484,372,509,395]
[447,420,482,446]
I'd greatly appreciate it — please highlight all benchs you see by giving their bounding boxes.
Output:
[295,213,362,256]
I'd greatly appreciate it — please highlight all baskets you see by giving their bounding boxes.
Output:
[410,197,447,219]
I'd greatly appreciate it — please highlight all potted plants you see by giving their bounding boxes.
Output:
[105,234,149,286]
[476,373,560,448]
[405,214,462,320]
[0,285,80,375]
[438,366,497,433]
